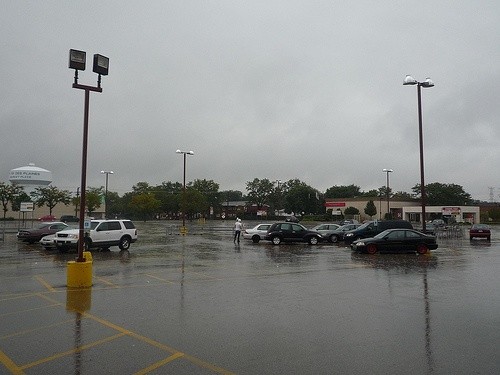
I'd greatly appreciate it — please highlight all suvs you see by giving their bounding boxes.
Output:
[264,222,323,245]
[53,219,138,253]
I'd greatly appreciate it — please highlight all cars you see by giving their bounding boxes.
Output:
[39,225,80,250]
[469,224,491,240]
[17,224,65,244]
[16,222,69,240]
[309,224,342,240]
[242,224,273,243]
[324,224,362,243]
[350,228,438,254]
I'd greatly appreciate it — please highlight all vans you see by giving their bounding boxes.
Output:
[343,220,413,245]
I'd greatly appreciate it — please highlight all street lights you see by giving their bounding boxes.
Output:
[100,170,114,221]
[66,48,109,289]
[402,74,435,234]
[176,149,194,235]
[383,169,393,213]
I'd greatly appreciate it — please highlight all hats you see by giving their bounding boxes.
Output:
[238,219,241,222]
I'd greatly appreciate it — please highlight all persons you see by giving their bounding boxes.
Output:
[233,218,242,243]
